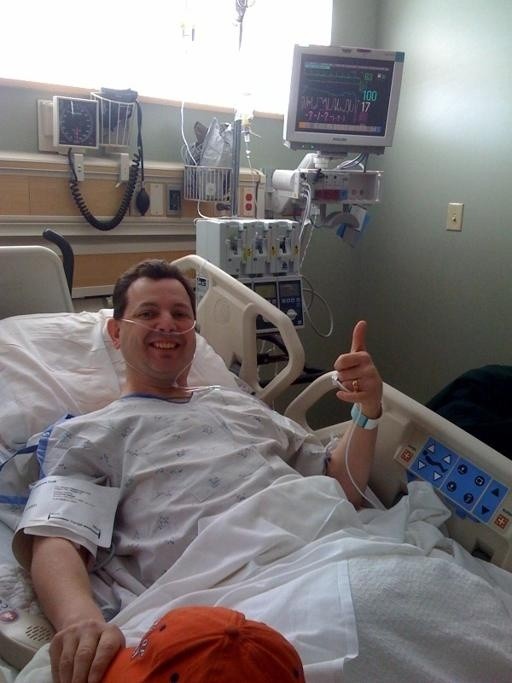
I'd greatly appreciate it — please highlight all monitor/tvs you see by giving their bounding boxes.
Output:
[282,44,405,154]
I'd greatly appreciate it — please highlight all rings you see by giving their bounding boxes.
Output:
[353,379,359,391]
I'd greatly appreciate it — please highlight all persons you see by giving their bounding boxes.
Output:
[13,256,388,682]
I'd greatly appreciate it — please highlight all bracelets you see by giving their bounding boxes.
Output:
[349,401,384,430]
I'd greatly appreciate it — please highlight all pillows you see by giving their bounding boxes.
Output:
[2,305,248,496]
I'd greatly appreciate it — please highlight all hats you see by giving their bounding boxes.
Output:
[96,604,307,682]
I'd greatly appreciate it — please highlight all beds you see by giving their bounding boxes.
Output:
[2,244,510,683]
